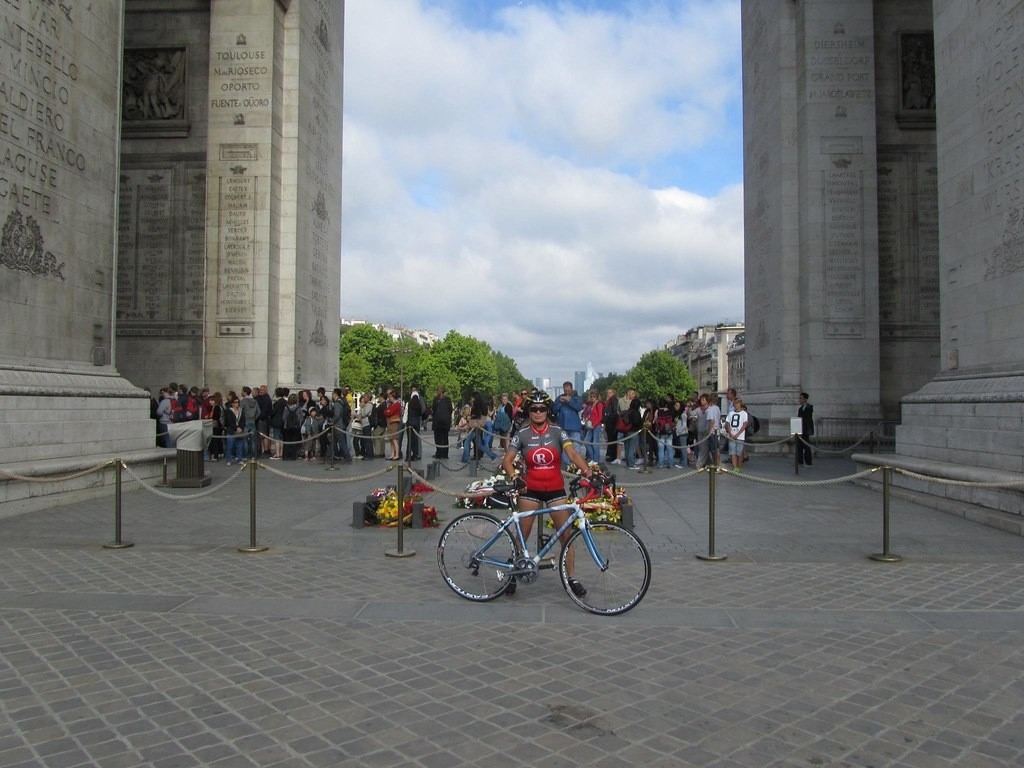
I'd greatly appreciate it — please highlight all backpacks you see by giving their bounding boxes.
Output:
[186,396,200,420]
[596,400,611,424]
[367,402,379,428]
[657,408,674,443]
[285,405,301,433]
[753,416,760,433]
[616,408,638,434]
[162,397,184,422]
[336,397,351,426]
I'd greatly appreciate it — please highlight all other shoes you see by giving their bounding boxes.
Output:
[587,460,599,467]
[257,454,318,461]
[238,462,244,465]
[798,463,813,470]
[610,459,683,469]
[386,455,423,462]
[698,464,742,473]
[321,453,385,465]
[225,460,231,465]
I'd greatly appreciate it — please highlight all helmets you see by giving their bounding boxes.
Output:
[524,390,553,411]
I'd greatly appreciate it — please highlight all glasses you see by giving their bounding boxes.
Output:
[591,395,596,398]
[521,392,528,395]
[527,406,548,412]
[234,401,239,404]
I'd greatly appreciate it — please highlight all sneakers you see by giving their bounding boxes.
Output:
[506,574,516,596]
[568,576,588,598]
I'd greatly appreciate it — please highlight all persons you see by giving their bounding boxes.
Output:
[455,381,755,473]
[795,392,814,468]
[501,391,602,598]
[432,387,453,460]
[143,382,426,466]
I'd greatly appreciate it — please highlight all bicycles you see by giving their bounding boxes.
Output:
[436,474,651,617]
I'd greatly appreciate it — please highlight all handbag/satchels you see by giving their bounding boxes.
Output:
[469,416,485,429]
[352,418,363,430]
[402,402,409,424]
[301,416,313,434]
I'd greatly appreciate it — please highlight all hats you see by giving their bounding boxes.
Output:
[309,407,316,415]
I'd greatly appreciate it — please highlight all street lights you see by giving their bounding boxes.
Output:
[391,345,413,401]
